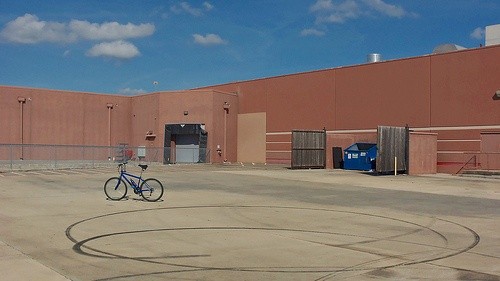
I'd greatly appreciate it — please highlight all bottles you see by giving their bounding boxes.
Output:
[130,179,137,187]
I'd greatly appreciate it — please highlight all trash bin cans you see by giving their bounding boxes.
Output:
[344,143,377,171]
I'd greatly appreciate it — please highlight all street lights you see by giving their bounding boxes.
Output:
[18,97,25,160]
[224,101,231,162]
[107,103,114,161]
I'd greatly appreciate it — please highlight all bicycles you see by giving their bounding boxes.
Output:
[104,161,164,202]
